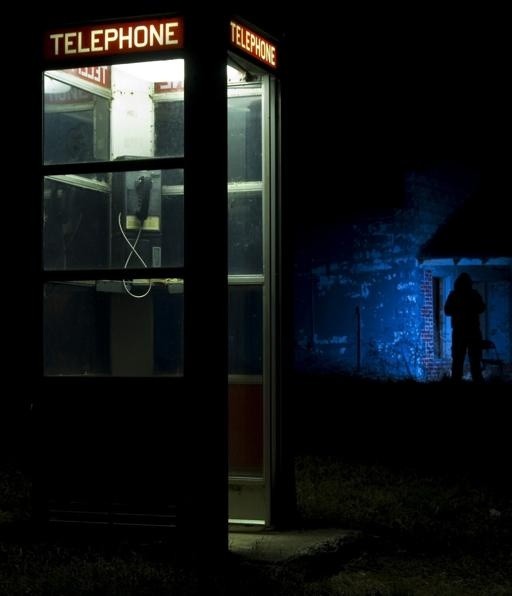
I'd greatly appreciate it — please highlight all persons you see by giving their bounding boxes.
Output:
[444,271,488,381]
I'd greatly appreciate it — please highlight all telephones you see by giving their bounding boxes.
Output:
[112,155,162,239]
